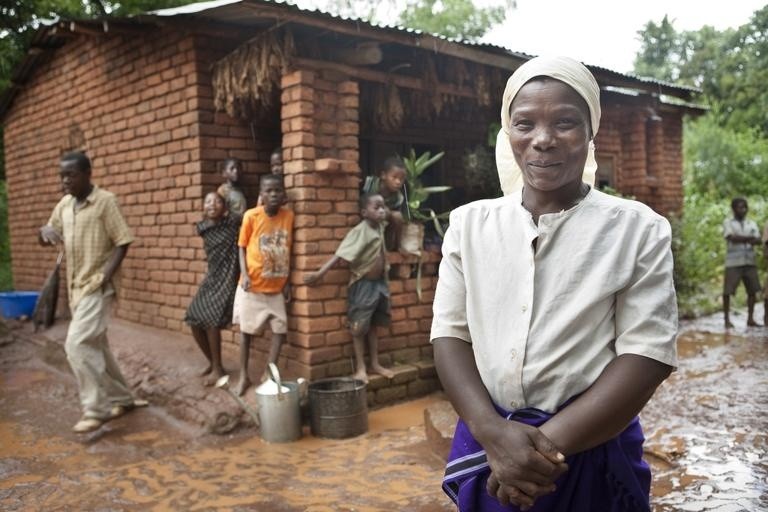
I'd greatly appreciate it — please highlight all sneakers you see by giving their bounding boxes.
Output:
[73,411,109,433]
[108,405,123,420]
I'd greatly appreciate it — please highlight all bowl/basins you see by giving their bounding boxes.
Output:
[0,291,39,320]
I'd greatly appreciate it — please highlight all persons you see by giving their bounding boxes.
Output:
[35,152,134,434]
[256,152,285,212]
[218,154,247,215]
[719,196,768,327]
[358,156,407,255]
[230,172,295,397]
[182,189,244,385]
[302,193,394,386]
[426,53,680,510]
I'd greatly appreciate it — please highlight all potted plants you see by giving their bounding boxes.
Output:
[396,148,449,256]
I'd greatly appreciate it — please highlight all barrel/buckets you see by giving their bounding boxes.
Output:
[307,377,370,441]
[253,361,304,443]
